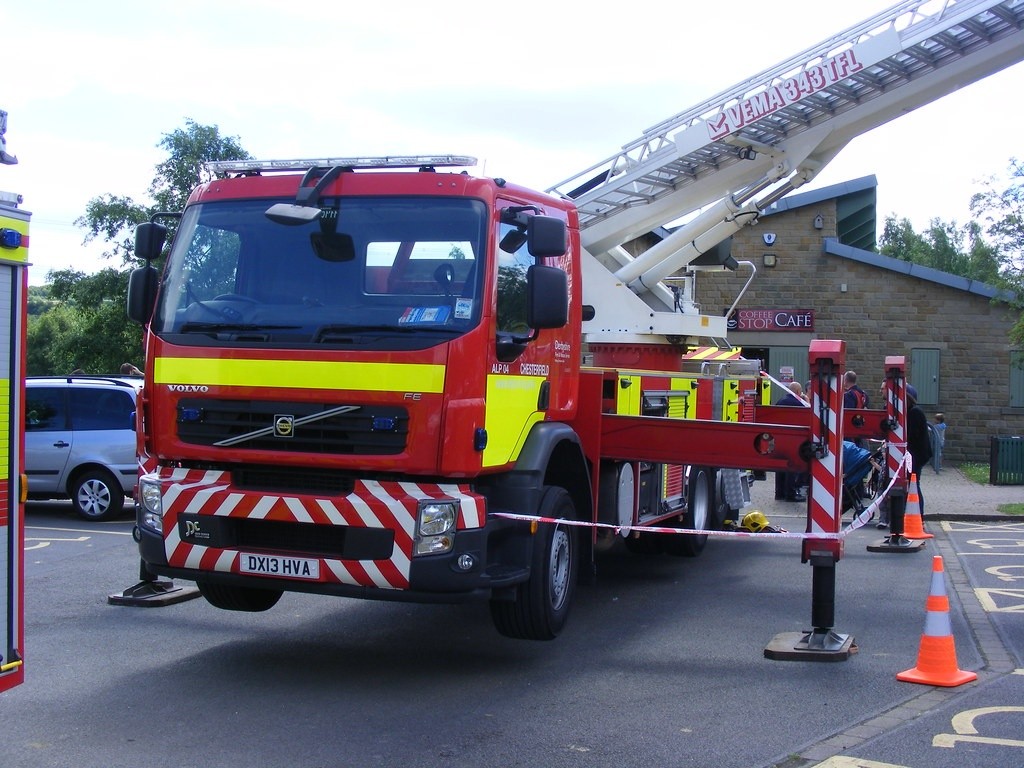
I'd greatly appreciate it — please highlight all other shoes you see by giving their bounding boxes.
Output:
[775,494,806,502]
[876,523,888,529]
[939,465,943,470]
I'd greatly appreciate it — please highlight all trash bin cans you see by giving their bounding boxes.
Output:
[989,435,1024,486]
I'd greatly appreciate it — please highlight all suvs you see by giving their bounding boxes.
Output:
[24,375,147,521]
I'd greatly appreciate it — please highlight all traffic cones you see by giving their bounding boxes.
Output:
[896,556,978,688]
[888,473,935,539]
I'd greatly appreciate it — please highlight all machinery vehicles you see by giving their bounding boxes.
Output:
[107,0,1024,660]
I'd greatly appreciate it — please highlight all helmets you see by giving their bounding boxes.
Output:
[741,511,769,533]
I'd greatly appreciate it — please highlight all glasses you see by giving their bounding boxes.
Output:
[880,387,887,390]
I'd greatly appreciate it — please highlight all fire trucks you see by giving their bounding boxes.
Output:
[0,110,32,693]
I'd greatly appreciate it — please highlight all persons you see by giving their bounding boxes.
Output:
[70,369,86,377]
[774,380,812,503]
[842,371,933,529]
[932,413,946,471]
[120,363,145,380]
